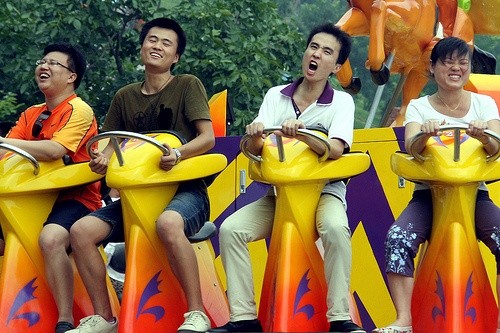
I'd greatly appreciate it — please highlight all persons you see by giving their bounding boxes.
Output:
[63,17,215,333]
[0,42,103,333]
[208,22,367,333]
[372,36,500,333]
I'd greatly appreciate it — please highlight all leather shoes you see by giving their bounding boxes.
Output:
[208,319,263,333]
[329,320,366,333]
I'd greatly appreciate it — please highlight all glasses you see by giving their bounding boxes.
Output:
[32,110,52,138]
[36,58,75,73]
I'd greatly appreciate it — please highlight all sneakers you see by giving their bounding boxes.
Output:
[65,314,118,333]
[176,310,211,333]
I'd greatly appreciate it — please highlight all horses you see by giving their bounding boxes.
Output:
[332,0,474,129]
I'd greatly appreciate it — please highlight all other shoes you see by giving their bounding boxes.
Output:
[371,326,412,333]
[55,320,74,333]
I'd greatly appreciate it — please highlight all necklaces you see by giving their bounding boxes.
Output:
[436,88,465,112]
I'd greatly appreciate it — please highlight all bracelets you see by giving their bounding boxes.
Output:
[482,136,493,147]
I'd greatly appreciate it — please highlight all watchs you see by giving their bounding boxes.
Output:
[172,148,182,165]
